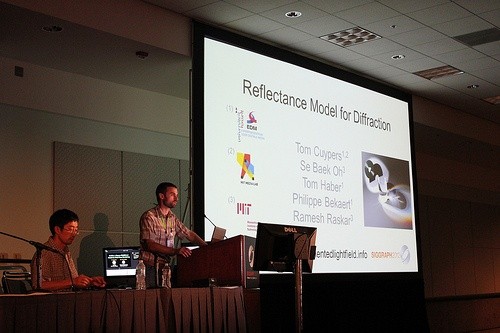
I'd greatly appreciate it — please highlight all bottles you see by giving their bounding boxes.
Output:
[161,263,172,288]
[135,259,146,290]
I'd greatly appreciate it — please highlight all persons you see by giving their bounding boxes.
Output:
[31,209,106,291]
[140,182,208,289]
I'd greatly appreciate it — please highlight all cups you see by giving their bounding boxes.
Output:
[15,253,22,259]
[2,252,8,260]
[208,278,216,287]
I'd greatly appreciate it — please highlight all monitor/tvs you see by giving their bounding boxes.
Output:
[253,223,318,272]
[103,246,141,283]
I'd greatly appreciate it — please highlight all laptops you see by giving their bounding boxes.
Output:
[211,227,226,242]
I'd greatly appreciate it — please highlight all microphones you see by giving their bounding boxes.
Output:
[202,214,227,239]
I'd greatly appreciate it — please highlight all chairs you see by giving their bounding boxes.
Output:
[2,272,33,293]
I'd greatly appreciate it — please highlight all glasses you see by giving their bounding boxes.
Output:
[60,226,79,235]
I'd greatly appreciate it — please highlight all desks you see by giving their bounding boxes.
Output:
[0,286,247,333]
